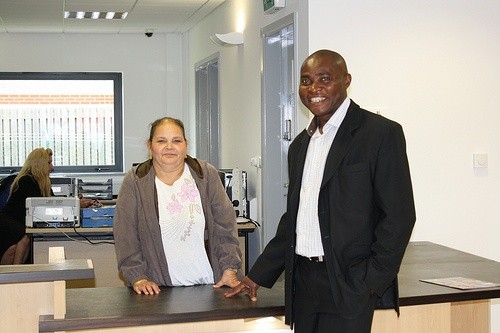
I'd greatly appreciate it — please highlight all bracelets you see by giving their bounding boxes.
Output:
[134,279,148,285]
[225,268,238,273]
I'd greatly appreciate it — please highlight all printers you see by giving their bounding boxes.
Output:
[25,197,80,228]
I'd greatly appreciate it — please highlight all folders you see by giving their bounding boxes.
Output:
[82,208,114,227]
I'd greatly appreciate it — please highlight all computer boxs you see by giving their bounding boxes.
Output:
[217,169,247,218]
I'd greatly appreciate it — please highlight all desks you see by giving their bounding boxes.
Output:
[0,241,500,333]
[25,214,256,277]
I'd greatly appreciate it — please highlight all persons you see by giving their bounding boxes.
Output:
[223,50,416,333]
[0,147,94,265]
[113,117,243,295]
